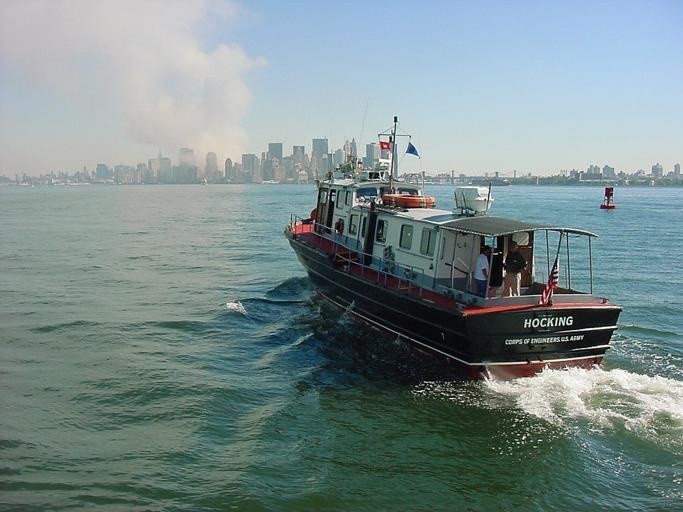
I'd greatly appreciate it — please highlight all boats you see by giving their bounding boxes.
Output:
[599,187,616,210]
[284,119,623,381]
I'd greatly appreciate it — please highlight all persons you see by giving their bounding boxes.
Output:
[472,245,491,299]
[502,241,527,296]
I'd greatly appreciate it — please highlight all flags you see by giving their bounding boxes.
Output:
[379,141,390,149]
[537,252,560,305]
[405,143,420,159]
